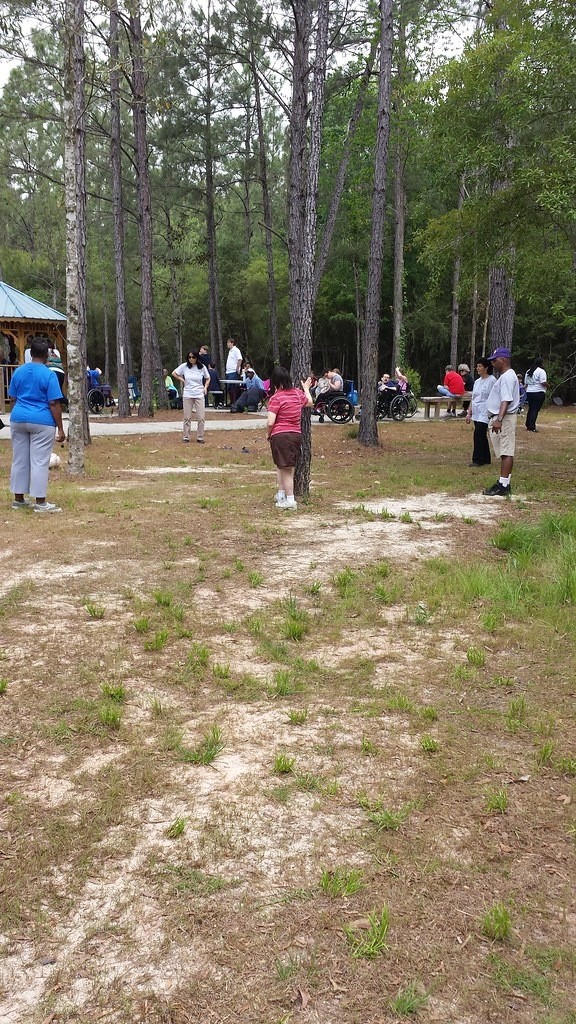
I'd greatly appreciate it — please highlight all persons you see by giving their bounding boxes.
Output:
[86,365,114,406]
[524,358,550,433]
[172,349,211,443]
[199,345,226,409]
[311,368,343,410]
[265,366,313,510]
[482,349,520,499]
[230,361,264,413]
[378,367,408,395]
[0,347,19,366]
[517,374,527,412]
[465,357,497,467]
[162,369,177,399]
[8,337,65,514]
[225,339,243,407]
[437,364,474,417]
[25,335,70,413]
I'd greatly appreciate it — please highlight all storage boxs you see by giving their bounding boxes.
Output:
[345,405,360,416]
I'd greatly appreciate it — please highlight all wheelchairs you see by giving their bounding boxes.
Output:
[376,382,418,422]
[87,370,116,414]
[310,380,354,424]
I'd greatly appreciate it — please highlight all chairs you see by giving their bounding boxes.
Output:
[259,379,271,412]
[127,375,141,409]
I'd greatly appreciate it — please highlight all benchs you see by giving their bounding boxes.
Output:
[205,391,223,406]
[421,396,471,421]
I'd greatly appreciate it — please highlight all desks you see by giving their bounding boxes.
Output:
[220,379,244,407]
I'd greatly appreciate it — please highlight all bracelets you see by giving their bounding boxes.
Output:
[497,416,503,422]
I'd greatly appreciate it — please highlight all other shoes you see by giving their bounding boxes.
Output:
[458,410,467,416]
[446,411,456,417]
[197,440,205,443]
[274,492,286,502]
[469,463,481,467]
[183,440,189,442]
[276,499,298,511]
[215,402,224,409]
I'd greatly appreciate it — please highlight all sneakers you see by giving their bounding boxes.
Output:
[12,498,36,509]
[34,502,61,512]
[483,480,511,495]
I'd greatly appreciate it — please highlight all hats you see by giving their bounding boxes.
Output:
[487,348,510,360]
[248,368,255,373]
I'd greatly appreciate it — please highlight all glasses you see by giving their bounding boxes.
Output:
[189,356,195,359]
